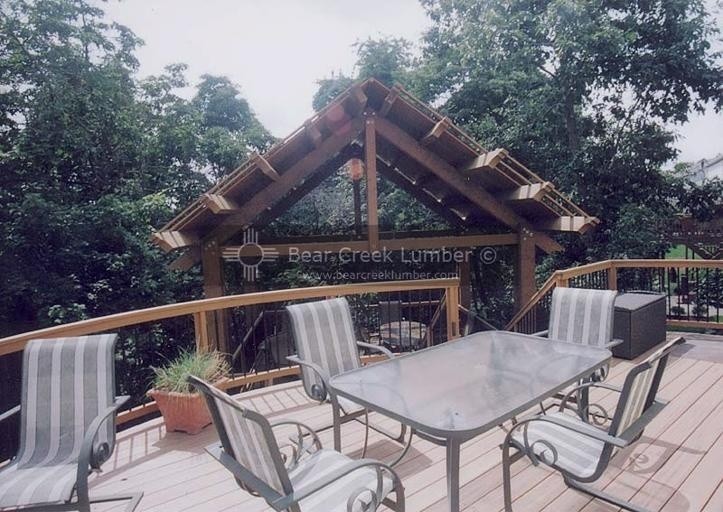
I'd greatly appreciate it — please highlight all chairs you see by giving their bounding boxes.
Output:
[0,284,685,512]
